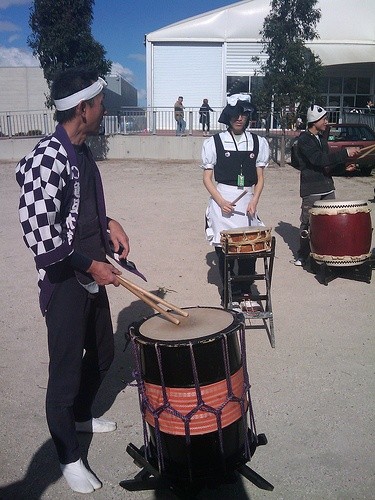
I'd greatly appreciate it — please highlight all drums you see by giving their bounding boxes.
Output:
[127,306,258,482]
[308,199,373,267]
[220,226,272,254]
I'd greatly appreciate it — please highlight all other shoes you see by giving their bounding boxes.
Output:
[228,302,242,312]
[295,255,309,265]
[239,294,262,311]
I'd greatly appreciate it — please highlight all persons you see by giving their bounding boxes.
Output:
[199,99,216,136]
[296,104,360,265]
[202,97,270,310]
[364,99,375,114]
[16,73,130,495]
[174,97,187,136]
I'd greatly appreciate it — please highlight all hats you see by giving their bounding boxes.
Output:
[218,93,258,129]
[307,103,328,123]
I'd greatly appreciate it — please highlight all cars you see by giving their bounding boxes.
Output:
[120,117,137,128]
[321,123,375,175]
